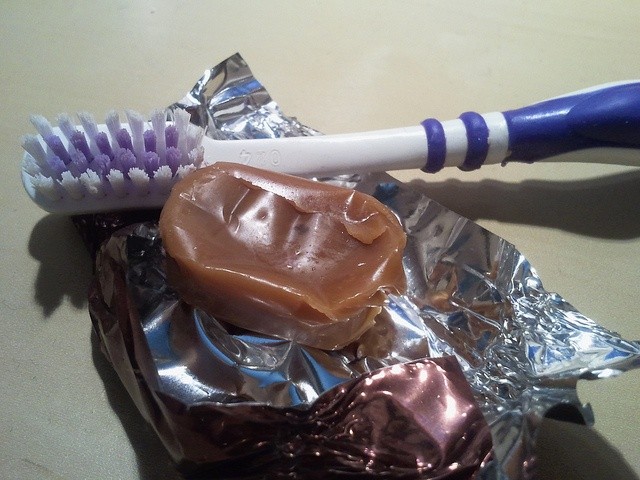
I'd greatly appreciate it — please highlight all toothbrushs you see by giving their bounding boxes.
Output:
[18,78,640,216]
